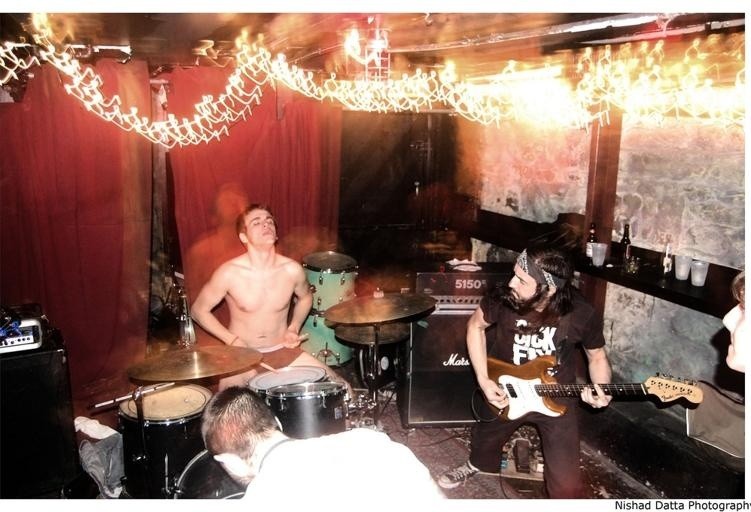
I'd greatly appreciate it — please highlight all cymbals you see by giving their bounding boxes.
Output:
[323,289,438,324]
[334,324,412,346]
[129,346,265,382]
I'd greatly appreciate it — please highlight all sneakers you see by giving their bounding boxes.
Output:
[438,459,480,489]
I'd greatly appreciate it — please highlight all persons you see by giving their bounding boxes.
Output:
[722,269,745,373]
[438,239,612,499]
[190,203,355,407]
[202,385,448,499]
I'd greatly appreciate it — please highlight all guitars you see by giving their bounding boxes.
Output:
[484,356,704,422]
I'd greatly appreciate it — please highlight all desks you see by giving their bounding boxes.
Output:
[470,209,745,321]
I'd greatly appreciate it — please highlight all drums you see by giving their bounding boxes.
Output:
[248,363,333,410]
[119,384,215,499]
[265,380,347,440]
[303,250,360,312]
[298,312,356,369]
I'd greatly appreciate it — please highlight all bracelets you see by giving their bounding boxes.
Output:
[230,336,239,346]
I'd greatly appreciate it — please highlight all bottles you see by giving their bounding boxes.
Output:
[657,233,675,278]
[621,225,632,261]
[586,223,600,266]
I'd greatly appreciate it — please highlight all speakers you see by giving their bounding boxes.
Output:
[397,271,511,430]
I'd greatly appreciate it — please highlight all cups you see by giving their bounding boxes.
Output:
[593,243,609,267]
[624,255,641,276]
[372,283,385,299]
[675,252,694,280]
[691,260,711,287]
[400,288,410,311]
